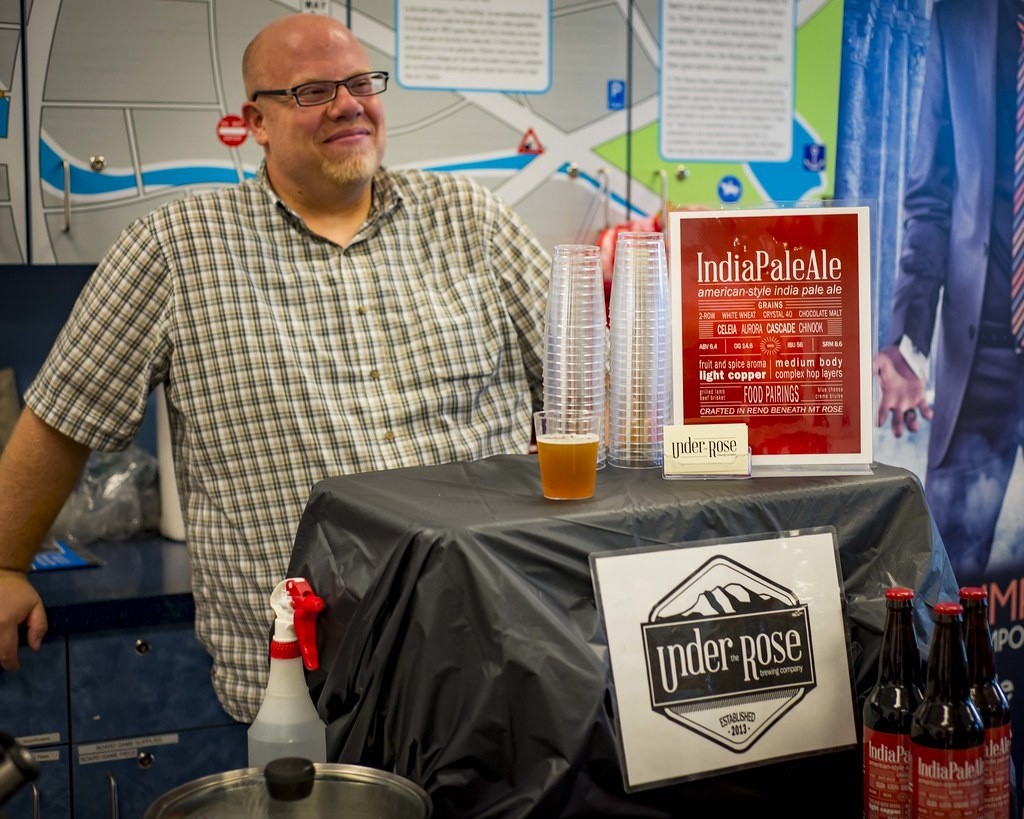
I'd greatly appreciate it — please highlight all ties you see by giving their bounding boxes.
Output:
[1009,14,1024,356]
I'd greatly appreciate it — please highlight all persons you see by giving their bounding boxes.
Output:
[0,15,611,722]
[872,0,1023,577]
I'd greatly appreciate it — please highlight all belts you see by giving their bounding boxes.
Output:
[978,325,1016,350]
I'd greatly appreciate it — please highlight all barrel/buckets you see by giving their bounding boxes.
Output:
[144,763,435,819]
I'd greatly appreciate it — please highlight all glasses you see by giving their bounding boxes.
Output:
[251,69,392,109]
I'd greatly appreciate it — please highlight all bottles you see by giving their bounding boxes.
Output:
[911,602,986,819]
[958,587,1011,819]
[258,757,319,819]
[861,587,926,819]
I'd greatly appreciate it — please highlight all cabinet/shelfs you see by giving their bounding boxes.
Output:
[0,593,249,819]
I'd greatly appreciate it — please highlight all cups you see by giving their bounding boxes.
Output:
[533,232,672,500]
